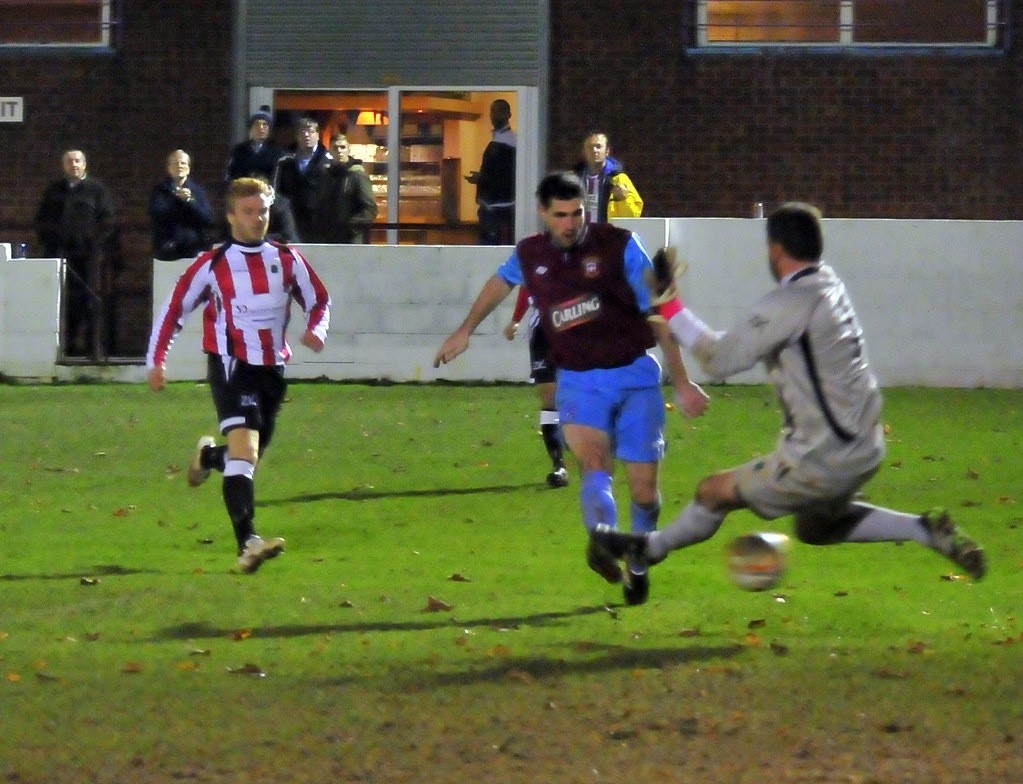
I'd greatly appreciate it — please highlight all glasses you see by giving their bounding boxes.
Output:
[297,128,317,134]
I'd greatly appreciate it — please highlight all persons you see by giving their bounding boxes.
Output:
[464,100,516,244]
[271,117,333,244]
[33,150,117,355]
[573,130,644,225]
[147,178,330,574]
[222,110,280,185]
[148,150,213,247]
[590,202,986,582]
[328,134,379,244]
[433,171,710,605]
[503,286,568,487]
[249,172,299,243]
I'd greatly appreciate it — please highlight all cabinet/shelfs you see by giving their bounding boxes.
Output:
[323,110,461,218]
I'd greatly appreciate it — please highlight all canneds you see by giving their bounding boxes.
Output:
[753,202,763,219]
[17,243,25,257]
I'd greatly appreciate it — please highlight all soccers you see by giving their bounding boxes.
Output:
[725,531,788,592]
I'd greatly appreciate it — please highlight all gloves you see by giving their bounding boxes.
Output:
[642,243,691,309]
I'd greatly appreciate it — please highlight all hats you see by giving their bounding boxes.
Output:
[249,103,274,125]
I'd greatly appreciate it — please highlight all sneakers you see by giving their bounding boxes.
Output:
[586,541,623,585]
[590,522,655,570]
[546,465,569,489]
[236,534,285,575]
[187,435,217,488]
[916,508,989,579]
[620,562,651,607]
